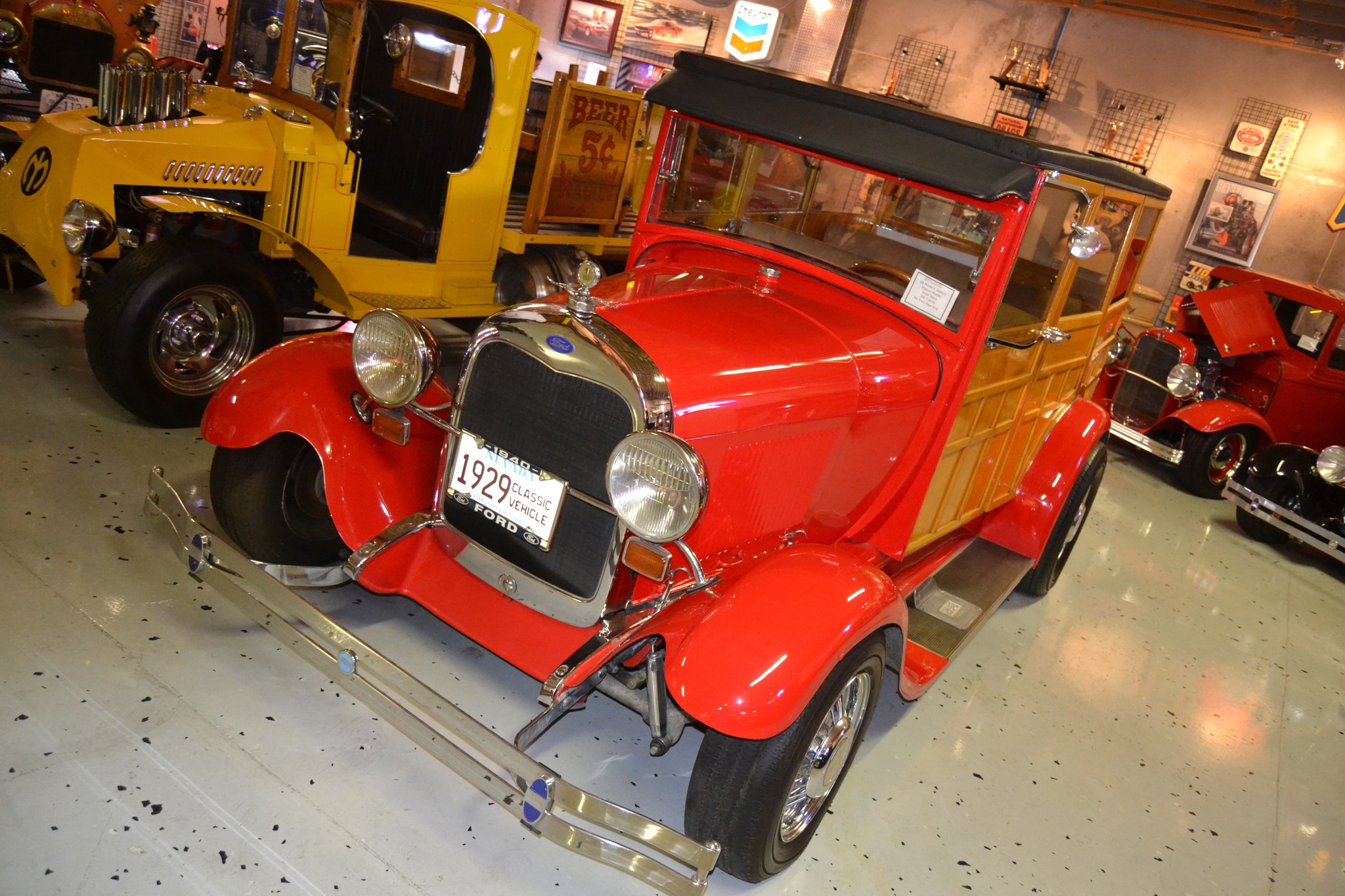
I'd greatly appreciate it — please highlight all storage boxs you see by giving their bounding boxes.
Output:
[1083,274,1165,340]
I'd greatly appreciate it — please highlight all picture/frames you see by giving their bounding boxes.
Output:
[1181,171,1283,268]
[179,0,206,45]
[557,0,625,60]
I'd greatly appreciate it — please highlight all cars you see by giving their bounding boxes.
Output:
[1103,263,1344,571]
[0,0,1173,894]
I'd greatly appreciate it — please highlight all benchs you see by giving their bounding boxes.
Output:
[347,84,463,235]
[819,220,1088,328]
